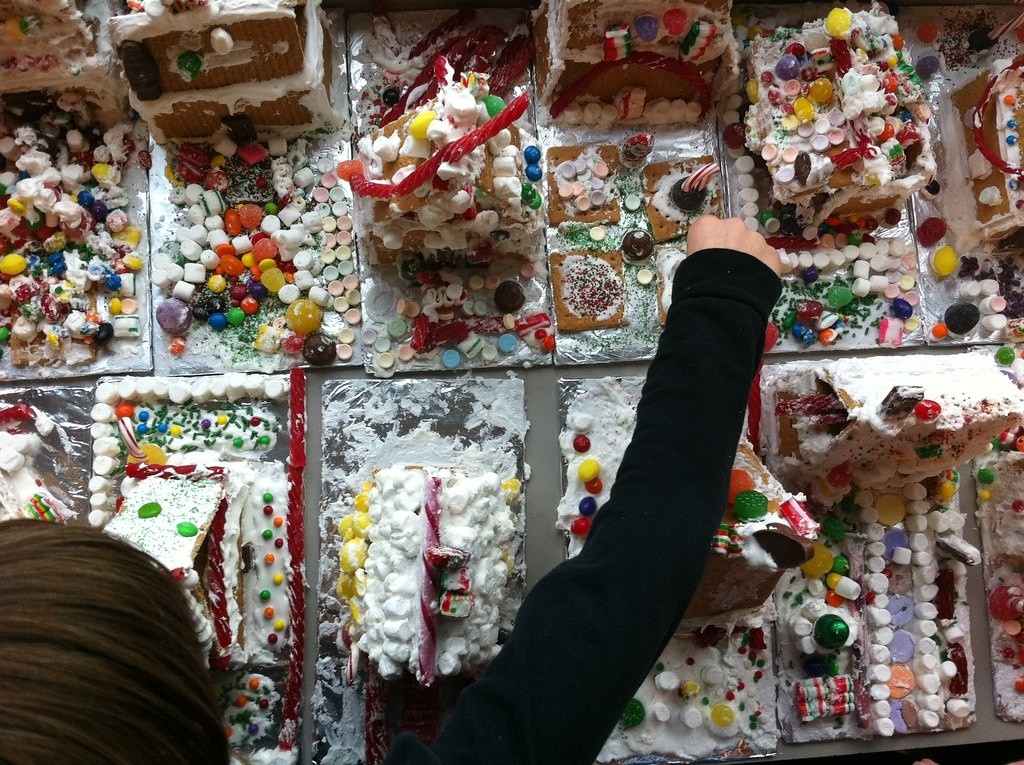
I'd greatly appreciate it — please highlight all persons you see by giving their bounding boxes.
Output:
[1,215,784,765]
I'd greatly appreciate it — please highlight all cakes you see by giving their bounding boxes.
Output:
[0,0,1024,765]
